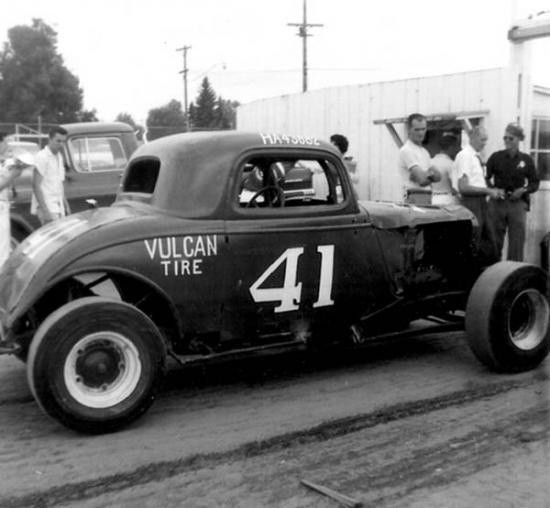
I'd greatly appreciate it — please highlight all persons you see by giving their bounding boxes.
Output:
[239,162,294,202]
[431,135,461,207]
[452,126,505,258]
[0,131,22,267]
[484,123,539,262]
[30,126,71,227]
[330,134,358,199]
[398,113,443,206]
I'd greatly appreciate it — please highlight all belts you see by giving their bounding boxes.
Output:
[409,191,431,194]
